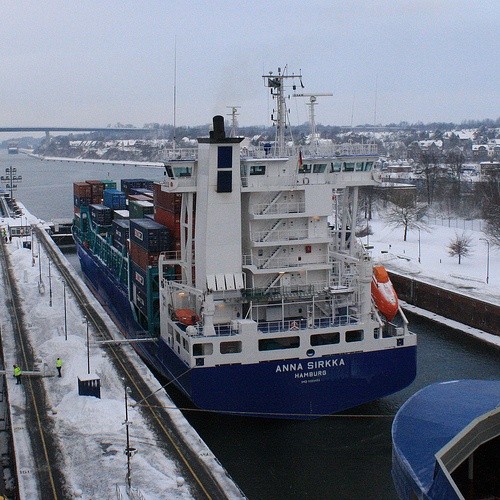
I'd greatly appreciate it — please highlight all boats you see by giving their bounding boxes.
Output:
[369,264,399,324]
[171,291,200,326]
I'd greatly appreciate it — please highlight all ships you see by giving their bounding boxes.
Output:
[41,64,419,428]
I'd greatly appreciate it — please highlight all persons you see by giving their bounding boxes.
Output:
[12,364,23,385]
[55,357,62,378]
[2,228,8,243]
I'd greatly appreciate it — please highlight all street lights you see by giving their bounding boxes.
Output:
[29,225,37,261]
[120,374,132,451]
[82,313,93,375]
[479,236,490,285]
[60,278,69,341]
[46,255,53,308]
